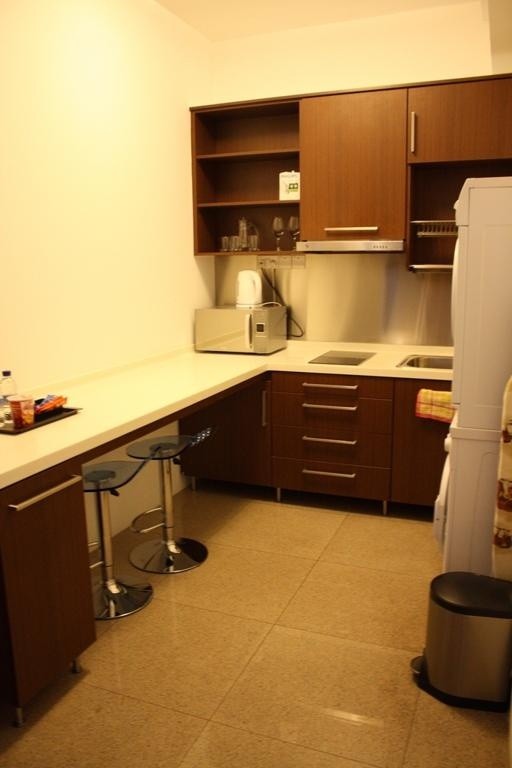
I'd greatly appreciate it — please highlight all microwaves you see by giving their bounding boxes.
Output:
[194,306,288,356]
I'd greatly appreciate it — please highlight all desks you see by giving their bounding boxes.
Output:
[0,339,290,491]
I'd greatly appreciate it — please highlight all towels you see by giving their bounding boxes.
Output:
[489,376,512,581]
[414,388,453,423]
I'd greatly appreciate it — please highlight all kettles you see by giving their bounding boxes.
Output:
[237,269,263,310]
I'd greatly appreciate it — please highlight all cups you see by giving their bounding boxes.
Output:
[6,394,34,428]
[287,216,300,249]
[247,235,258,252]
[272,217,284,252]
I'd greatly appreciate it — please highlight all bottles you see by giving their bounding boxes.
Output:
[0,371,18,424]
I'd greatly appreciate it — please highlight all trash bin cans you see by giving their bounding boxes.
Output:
[411,570,512,714]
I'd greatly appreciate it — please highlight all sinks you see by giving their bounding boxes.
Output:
[397,353,453,370]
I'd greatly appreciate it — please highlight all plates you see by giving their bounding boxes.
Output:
[0,407,77,434]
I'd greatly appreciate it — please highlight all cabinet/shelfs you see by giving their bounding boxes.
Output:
[406,73,512,275]
[178,372,272,488]
[390,378,452,507]
[270,373,394,505]
[0,465,98,710]
[190,84,408,257]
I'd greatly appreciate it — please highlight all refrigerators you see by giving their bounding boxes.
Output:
[434,176,512,577]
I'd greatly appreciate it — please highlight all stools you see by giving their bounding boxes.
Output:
[82,445,161,621]
[126,428,212,574]
